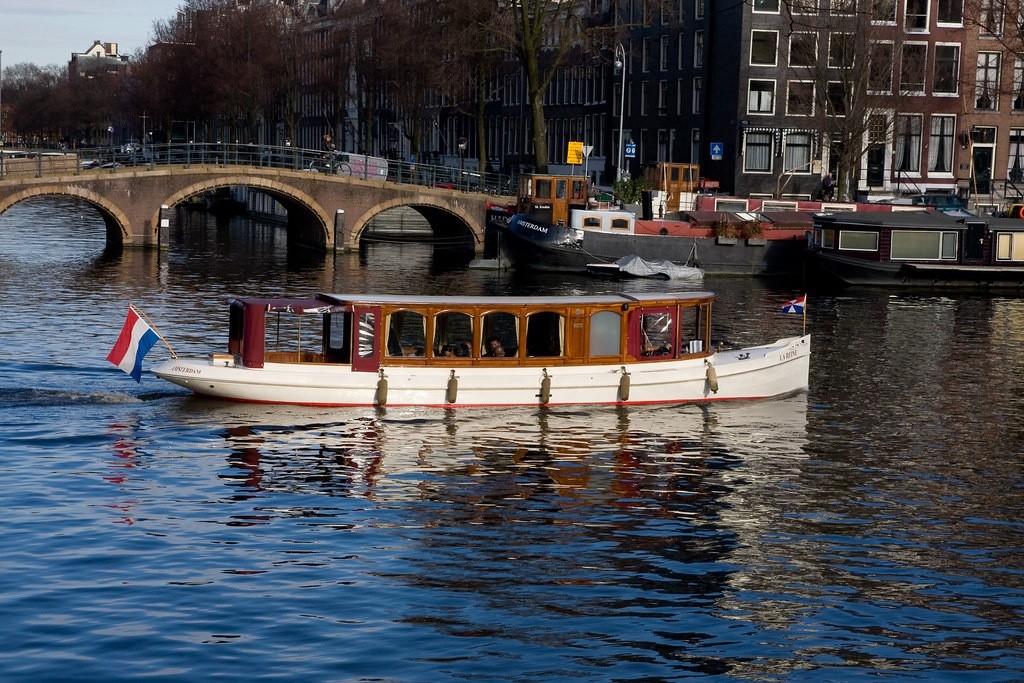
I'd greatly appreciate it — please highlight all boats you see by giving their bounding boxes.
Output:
[146,288,814,405]
[810,204,1024,286]
[586,255,704,281]
[502,213,816,272]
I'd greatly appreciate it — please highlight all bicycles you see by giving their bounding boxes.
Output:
[309,150,352,179]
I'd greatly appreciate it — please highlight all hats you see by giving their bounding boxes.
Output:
[454,343,469,356]
[323,134,331,140]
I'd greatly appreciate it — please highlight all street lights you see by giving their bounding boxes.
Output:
[613,42,626,182]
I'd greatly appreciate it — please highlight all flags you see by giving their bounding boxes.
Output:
[781,294,807,314]
[106,306,161,384]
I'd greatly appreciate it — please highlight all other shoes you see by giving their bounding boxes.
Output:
[325,164,330,168]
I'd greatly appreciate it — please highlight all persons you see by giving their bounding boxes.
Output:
[321,134,335,167]
[407,338,505,358]
[710,339,716,353]
[822,172,836,197]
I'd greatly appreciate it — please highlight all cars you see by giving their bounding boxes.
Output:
[904,194,976,219]
